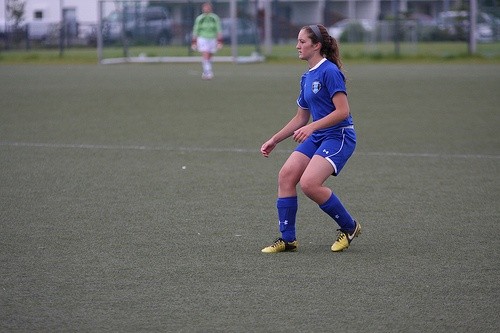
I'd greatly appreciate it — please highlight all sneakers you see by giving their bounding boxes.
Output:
[261,237,297,253]
[330,221,362,252]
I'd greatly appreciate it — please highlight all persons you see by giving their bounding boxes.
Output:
[260,26,361,254]
[191,3,222,78]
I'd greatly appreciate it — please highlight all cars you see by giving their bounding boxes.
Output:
[183,17,263,44]
[328,17,372,42]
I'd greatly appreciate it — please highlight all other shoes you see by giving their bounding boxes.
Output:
[202,72,207,79]
[207,72,213,80]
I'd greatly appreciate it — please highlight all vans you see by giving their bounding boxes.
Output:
[103,8,170,46]
